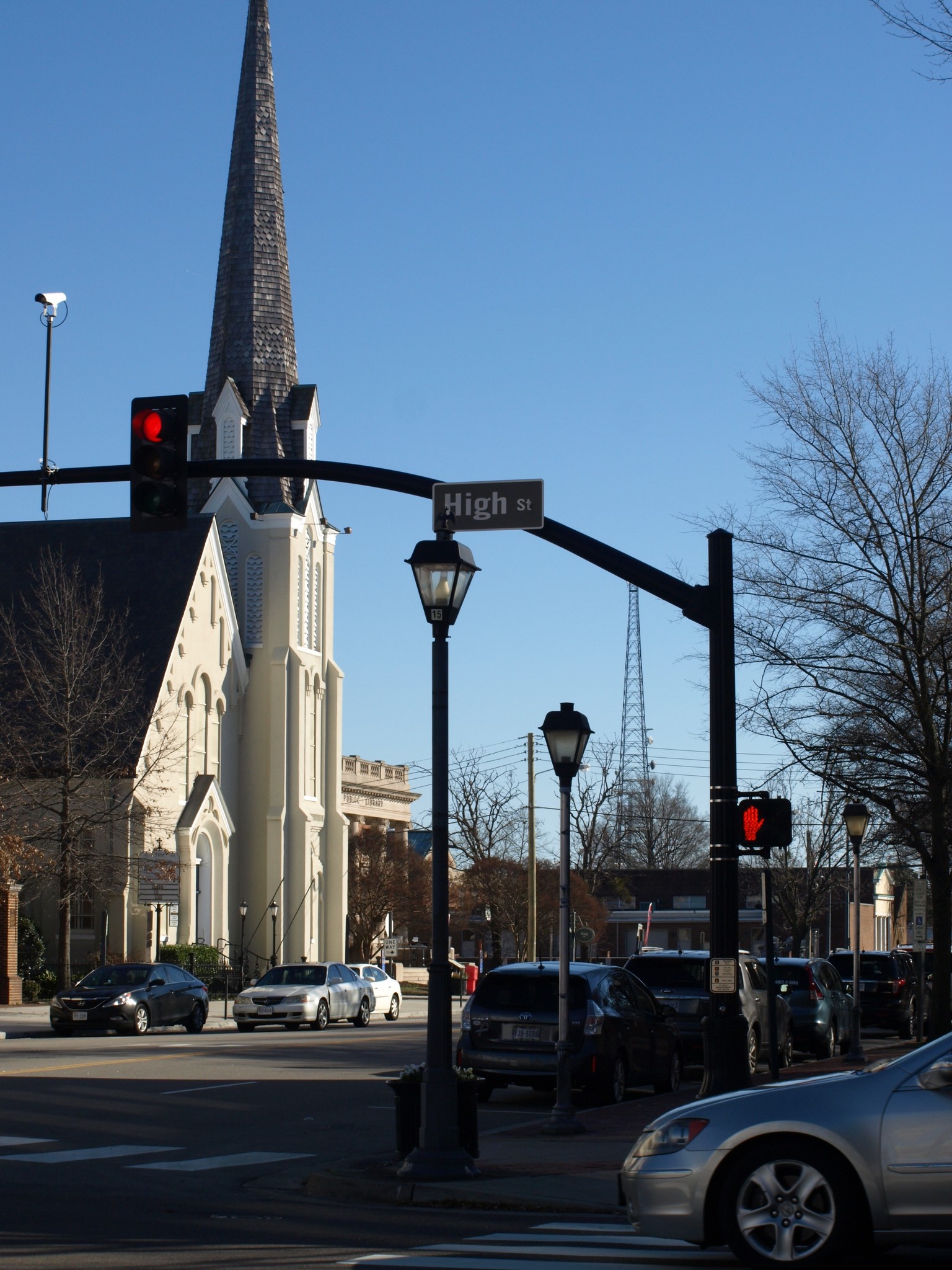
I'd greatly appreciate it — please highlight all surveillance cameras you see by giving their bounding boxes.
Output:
[34,289,67,306]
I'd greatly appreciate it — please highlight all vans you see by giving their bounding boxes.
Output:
[895,943,937,1026]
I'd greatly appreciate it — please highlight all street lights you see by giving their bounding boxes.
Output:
[238,899,243,993]
[540,701,596,1137]
[402,518,482,1186]
[839,795,872,1065]
[269,898,279,968]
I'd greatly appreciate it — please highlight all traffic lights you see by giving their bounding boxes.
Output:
[127,393,190,534]
[726,787,796,860]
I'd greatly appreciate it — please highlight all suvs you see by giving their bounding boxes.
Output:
[619,948,794,1078]
[822,947,933,1042]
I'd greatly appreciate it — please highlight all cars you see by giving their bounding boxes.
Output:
[615,1028,952,1265]
[336,964,399,1022]
[743,955,859,1057]
[50,963,211,1035]
[234,961,375,1030]
[452,962,682,1110]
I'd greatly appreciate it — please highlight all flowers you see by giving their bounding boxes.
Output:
[401,1062,477,1081]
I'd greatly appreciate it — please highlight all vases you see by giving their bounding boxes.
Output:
[385,1077,491,1183]
